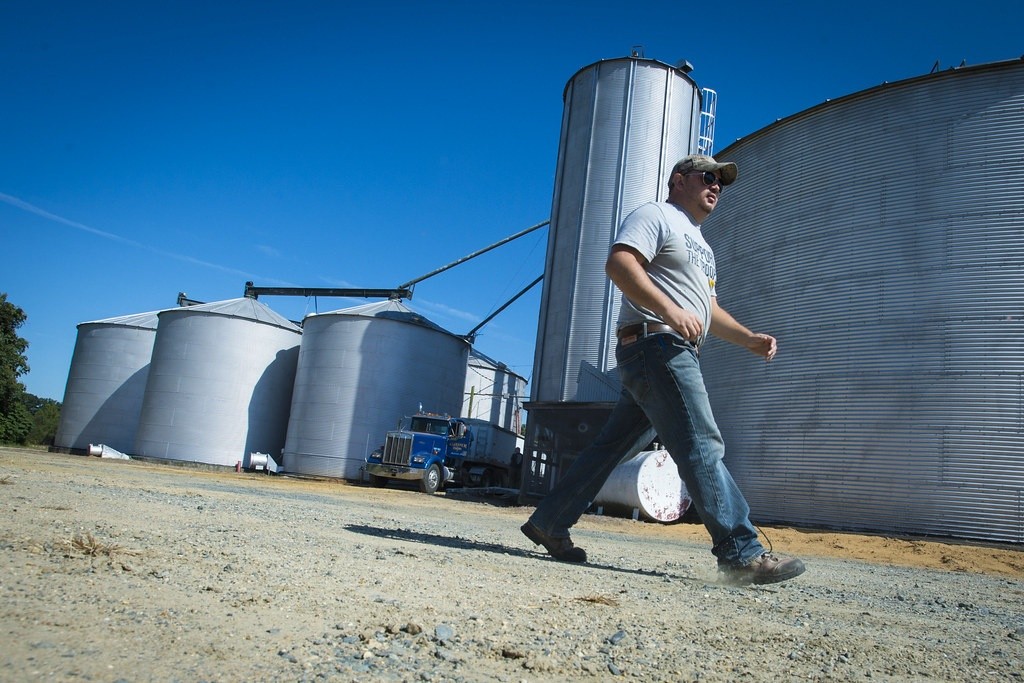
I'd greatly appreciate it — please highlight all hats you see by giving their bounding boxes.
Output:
[668,154,739,187]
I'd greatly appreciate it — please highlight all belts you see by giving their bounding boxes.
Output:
[618,323,700,358]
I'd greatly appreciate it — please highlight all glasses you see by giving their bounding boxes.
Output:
[685,171,724,193]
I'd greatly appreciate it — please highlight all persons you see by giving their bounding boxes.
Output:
[520,154,807,586]
[464,428,474,449]
[510,448,523,489]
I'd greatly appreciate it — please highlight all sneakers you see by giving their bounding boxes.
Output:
[521,520,587,561]
[724,550,805,584]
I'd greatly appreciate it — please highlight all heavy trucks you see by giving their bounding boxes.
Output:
[361,402,526,497]
